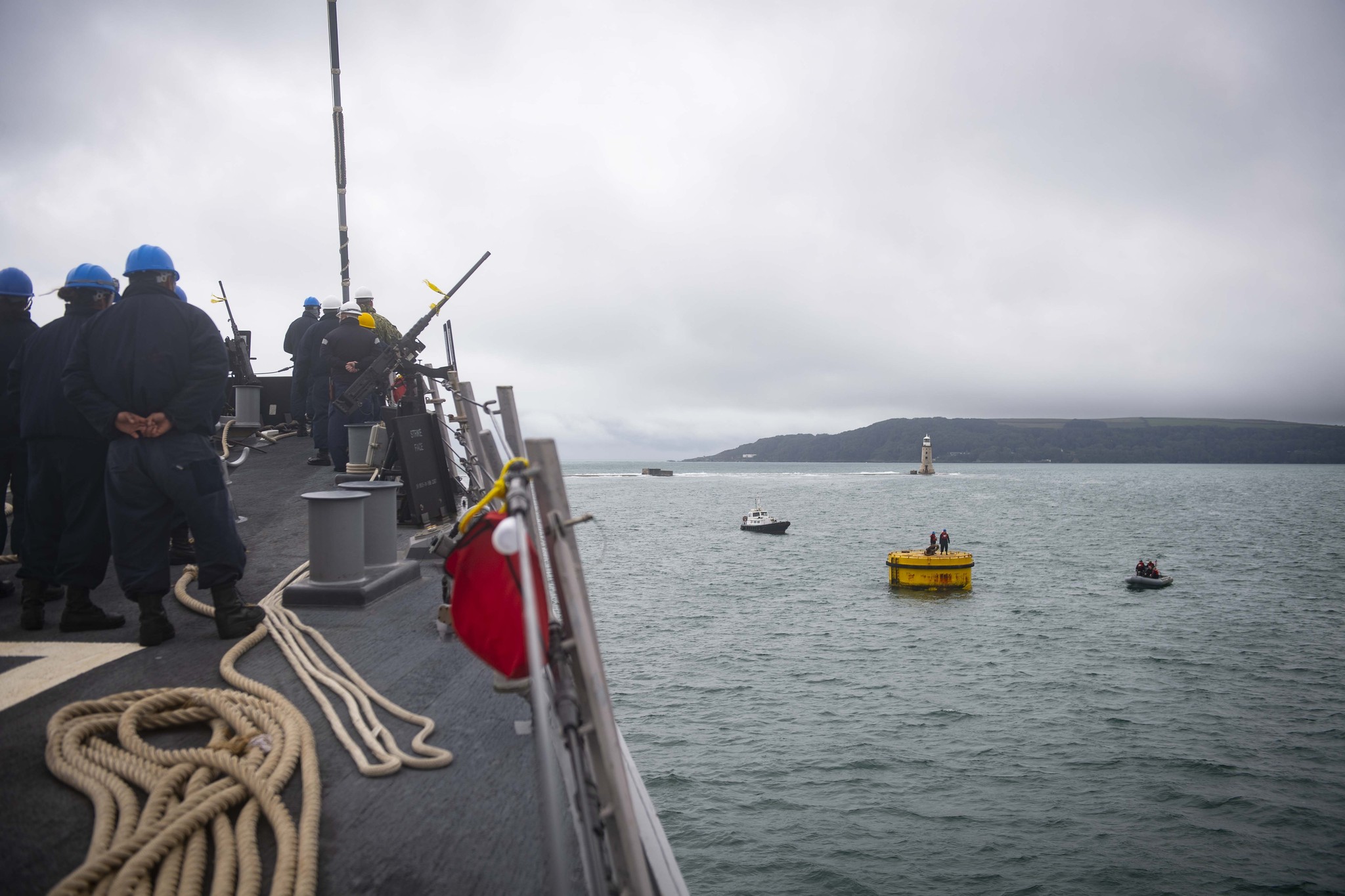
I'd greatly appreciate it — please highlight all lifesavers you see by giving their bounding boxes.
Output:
[742,516,747,521]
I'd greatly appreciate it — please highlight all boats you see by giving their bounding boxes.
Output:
[641,468,673,476]
[740,493,791,535]
[1122,575,1174,587]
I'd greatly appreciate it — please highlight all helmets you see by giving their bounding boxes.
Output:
[56,264,120,302]
[303,297,320,306]
[322,297,341,310]
[0,268,34,296]
[123,244,180,281]
[174,284,187,303]
[358,312,376,329]
[336,301,363,318]
[354,286,374,300]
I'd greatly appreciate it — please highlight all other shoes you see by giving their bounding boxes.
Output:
[212,604,265,638]
[18,612,45,630]
[136,615,175,645]
[0,580,17,600]
[297,428,313,437]
[58,607,124,631]
[333,467,346,472]
[167,546,198,564]
[307,455,331,466]
[48,587,65,601]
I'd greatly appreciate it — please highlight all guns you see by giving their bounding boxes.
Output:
[219,280,263,384]
[331,250,491,418]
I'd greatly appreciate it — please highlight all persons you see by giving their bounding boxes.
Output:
[939,529,950,555]
[283,286,402,473]
[61,243,266,646]
[0,263,196,632]
[931,531,937,554]
[1135,560,1159,579]
